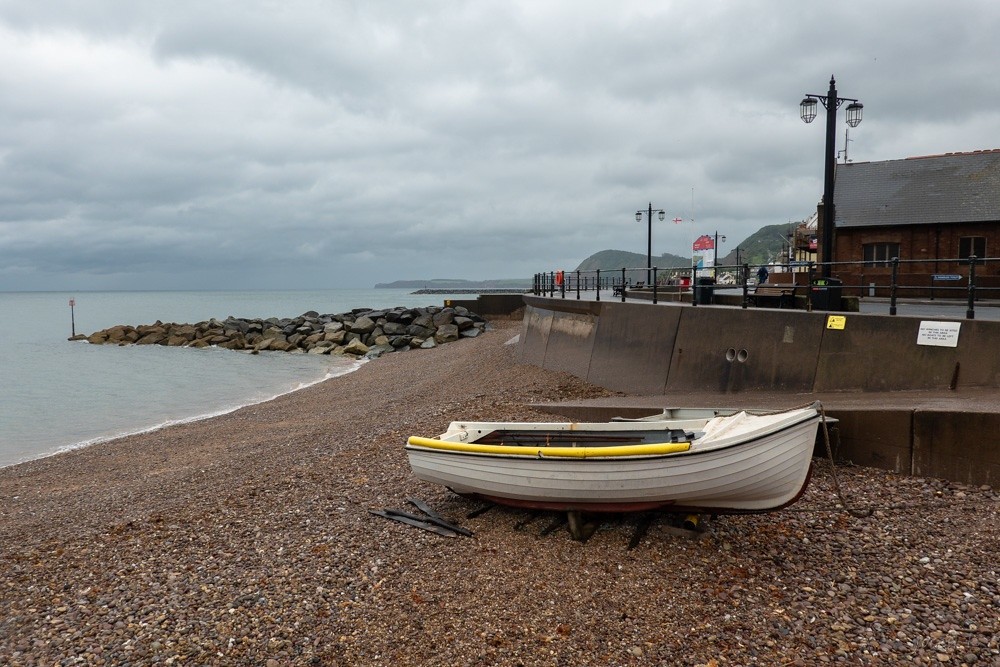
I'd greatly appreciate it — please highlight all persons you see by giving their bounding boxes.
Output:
[756,264,769,284]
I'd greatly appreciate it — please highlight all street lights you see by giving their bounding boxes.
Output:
[705,231,727,284]
[799,74,864,277]
[731,246,745,284]
[635,202,665,287]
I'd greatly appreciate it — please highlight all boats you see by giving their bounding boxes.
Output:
[405,397,839,516]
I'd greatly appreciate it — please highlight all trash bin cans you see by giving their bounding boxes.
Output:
[679,276,690,289]
[810,276,843,311]
[694,276,717,304]
